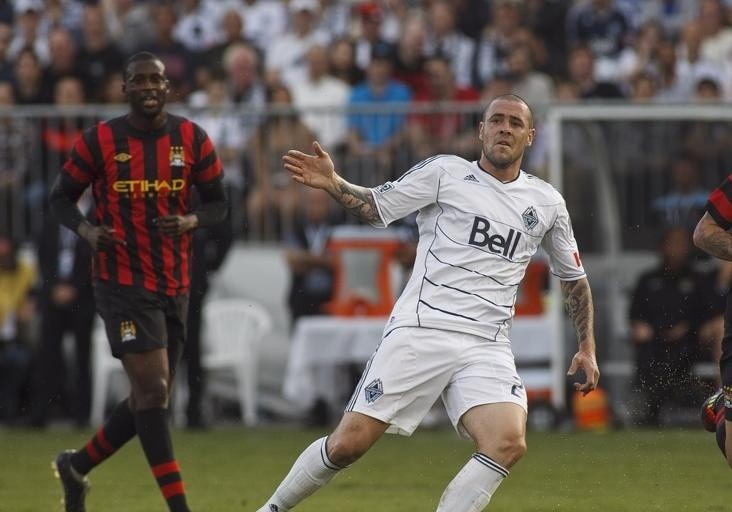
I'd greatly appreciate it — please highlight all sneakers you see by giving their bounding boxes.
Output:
[701,393,724,433]
[51,449,92,512]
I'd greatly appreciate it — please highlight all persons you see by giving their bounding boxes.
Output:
[693,165,731,471]
[253,93,602,511]
[1,0,731,433]
[48,51,232,512]
